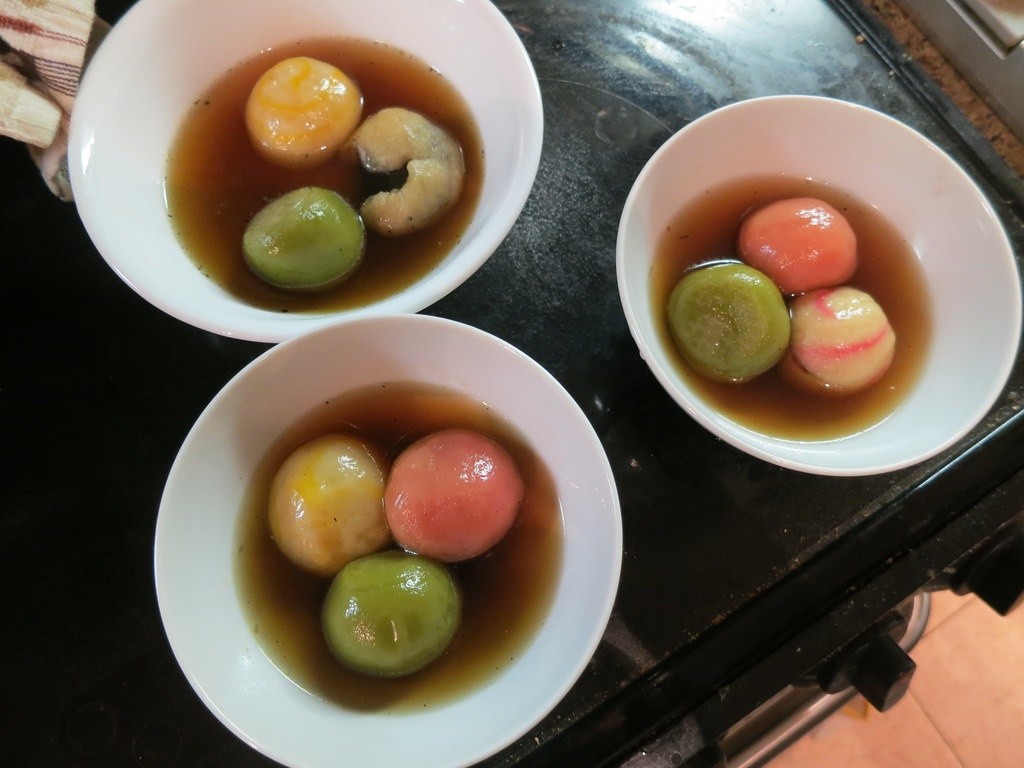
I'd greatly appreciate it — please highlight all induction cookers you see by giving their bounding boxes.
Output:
[0,0,1023,768]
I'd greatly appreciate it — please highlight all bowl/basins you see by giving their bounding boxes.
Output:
[65,0,546,340]
[616,94,1023,478]
[152,315,623,768]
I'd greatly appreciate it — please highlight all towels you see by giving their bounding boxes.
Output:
[0,2,116,200]
